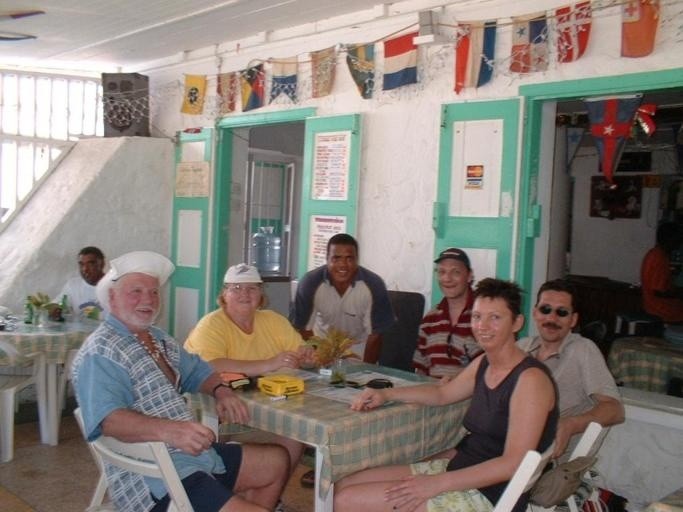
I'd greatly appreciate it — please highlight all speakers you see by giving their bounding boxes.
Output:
[100,72,150,136]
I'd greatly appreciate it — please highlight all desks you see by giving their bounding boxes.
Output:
[587,387,683,512]
[563,274,645,340]
[201,358,473,512]
[607,336,683,396]
[0,315,104,445]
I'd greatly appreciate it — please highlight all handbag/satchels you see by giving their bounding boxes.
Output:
[527,453,599,509]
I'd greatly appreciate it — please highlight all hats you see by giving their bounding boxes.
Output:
[108,250,177,288]
[433,246,469,263]
[221,261,264,284]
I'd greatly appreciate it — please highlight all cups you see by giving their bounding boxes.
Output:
[331,357,347,387]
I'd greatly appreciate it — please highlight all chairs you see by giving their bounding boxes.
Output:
[74,407,197,512]
[536,420,611,512]
[0,334,50,463]
[491,450,542,512]
[57,348,78,428]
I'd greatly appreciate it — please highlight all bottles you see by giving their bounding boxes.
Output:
[22,292,35,325]
[59,293,70,321]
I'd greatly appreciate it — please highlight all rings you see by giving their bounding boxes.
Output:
[285,358,288,361]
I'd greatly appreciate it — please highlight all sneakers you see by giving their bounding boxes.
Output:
[300,469,315,490]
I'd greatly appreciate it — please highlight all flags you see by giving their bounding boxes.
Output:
[216,74,237,112]
[555,0,593,63]
[268,56,298,106]
[346,44,375,100]
[511,11,550,75]
[454,22,496,92]
[310,46,338,98]
[383,32,418,91]
[240,64,264,111]
[621,0,659,57]
[180,76,206,113]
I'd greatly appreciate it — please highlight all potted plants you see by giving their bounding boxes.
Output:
[305,323,363,388]
[28,291,51,326]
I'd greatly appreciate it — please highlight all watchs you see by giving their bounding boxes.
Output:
[213,383,232,396]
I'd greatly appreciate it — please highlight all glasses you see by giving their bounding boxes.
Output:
[226,285,264,293]
[536,304,573,318]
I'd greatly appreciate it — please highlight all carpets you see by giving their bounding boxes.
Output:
[0,414,109,512]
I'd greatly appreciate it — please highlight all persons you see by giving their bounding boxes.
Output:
[288,232,396,488]
[413,248,485,460]
[517,280,626,505]
[332,278,560,512]
[183,263,318,507]
[70,251,290,511]
[54,247,106,320]
